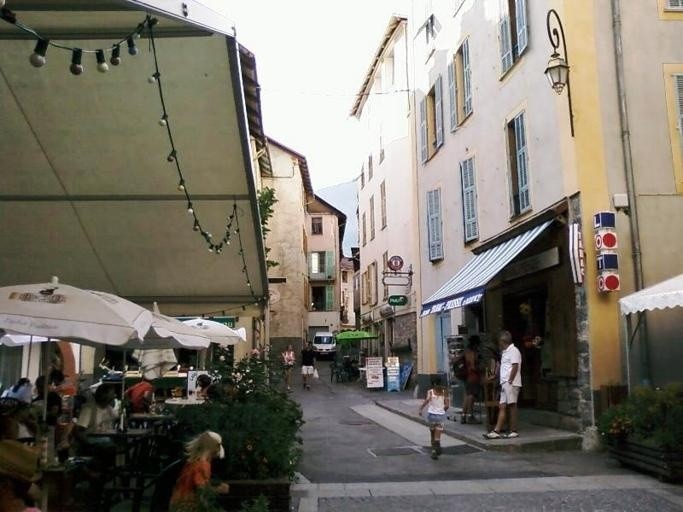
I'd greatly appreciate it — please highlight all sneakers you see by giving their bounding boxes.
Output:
[466,415,480,423]
[482,430,501,439]
[430,447,438,459]
[437,449,441,455]
[502,430,519,438]
[460,415,466,424]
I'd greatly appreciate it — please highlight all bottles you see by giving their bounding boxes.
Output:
[149,392,156,416]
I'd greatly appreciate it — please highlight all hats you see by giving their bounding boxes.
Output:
[468,335,482,344]
[0,439,43,483]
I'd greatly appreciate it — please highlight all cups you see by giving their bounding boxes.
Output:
[171,390,177,400]
[55,443,72,464]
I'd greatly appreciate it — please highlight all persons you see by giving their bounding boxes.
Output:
[0,351,156,512]
[281,344,296,391]
[487,347,506,433]
[461,334,482,424]
[418,375,449,459]
[483,332,522,439]
[300,341,320,392]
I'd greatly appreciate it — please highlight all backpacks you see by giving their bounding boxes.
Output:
[453,357,467,381]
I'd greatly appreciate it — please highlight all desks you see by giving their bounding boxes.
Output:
[123,370,188,397]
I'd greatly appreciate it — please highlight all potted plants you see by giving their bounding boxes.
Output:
[597,382,683,482]
[154,350,305,511]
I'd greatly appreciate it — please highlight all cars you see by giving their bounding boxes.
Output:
[311,331,337,360]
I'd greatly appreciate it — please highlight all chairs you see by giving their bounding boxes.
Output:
[0,383,211,512]
[329,361,362,383]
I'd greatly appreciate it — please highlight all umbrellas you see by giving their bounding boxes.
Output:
[335,329,379,370]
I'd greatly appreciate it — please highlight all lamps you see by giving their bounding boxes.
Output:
[546,9,575,140]
